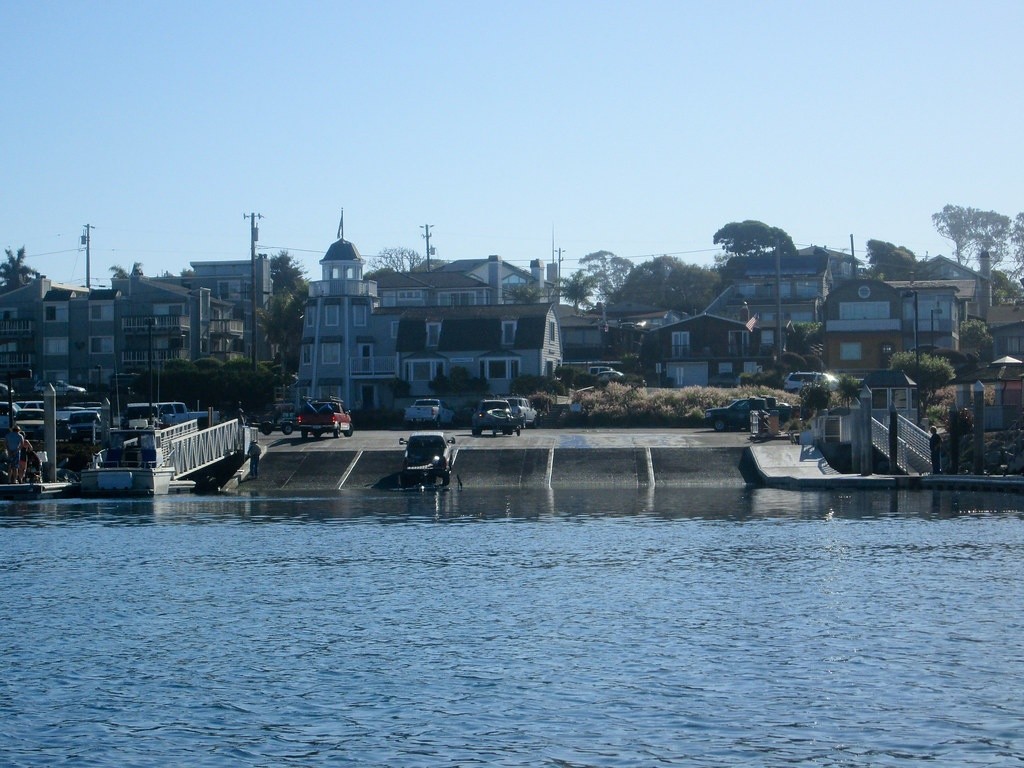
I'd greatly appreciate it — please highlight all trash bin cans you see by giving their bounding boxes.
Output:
[42,462,51,483]
[768,410,779,435]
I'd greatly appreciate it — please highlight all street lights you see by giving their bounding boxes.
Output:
[95,365,103,388]
[900,290,924,429]
[5,369,32,433]
[143,317,159,429]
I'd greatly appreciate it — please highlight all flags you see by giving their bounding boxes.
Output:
[745,312,760,332]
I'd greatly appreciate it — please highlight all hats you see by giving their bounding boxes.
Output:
[252,440,257,444]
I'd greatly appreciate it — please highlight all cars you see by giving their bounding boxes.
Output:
[588,366,647,388]
[0,399,104,443]
[34,380,88,396]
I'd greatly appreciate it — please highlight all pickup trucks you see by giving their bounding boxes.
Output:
[157,402,220,427]
[704,398,804,431]
[403,398,457,428]
[298,396,354,438]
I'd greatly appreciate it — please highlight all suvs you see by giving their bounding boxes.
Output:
[397,432,456,486]
[470,399,514,437]
[783,371,840,394]
[503,396,540,429]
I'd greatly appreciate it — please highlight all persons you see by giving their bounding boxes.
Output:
[3,425,42,484]
[247,440,261,479]
[930,426,943,474]
